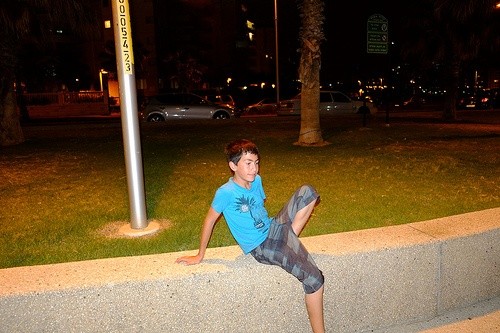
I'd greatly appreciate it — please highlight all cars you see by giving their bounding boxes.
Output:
[246,97,278,110]
[141,91,239,123]
[352,75,500,111]
[277,90,377,115]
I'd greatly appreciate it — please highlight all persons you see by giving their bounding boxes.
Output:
[175,139,326,333]
